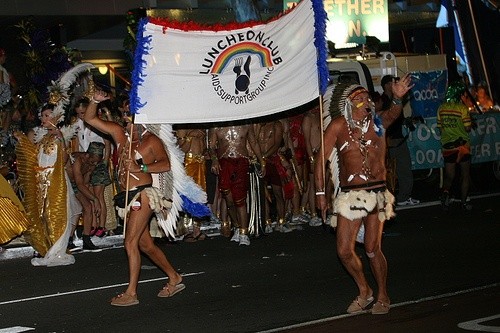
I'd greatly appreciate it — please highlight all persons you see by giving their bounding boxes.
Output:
[379,74,419,205]
[315,72,415,314]
[437,81,500,209]
[12,104,81,258]
[86,91,184,306]
[0,51,17,176]
[70,98,334,252]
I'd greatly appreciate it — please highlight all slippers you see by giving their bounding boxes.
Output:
[157,283,185,298]
[347,296,374,314]
[111,292,139,307]
[372,299,390,315]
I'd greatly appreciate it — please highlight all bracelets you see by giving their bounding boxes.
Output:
[93,99,100,103]
[316,192,325,195]
[392,97,401,104]
[142,163,147,173]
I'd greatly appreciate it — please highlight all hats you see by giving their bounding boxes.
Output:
[381,74,400,88]
[88,142,105,154]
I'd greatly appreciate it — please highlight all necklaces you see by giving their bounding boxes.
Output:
[125,128,148,142]
[346,122,364,142]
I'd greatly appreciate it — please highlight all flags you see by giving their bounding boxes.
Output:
[131,0,321,125]
[436,0,473,85]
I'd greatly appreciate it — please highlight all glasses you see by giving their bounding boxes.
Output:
[354,96,372,102]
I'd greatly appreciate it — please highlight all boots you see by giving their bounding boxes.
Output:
[82,234,103,253]
[67,235,83,253]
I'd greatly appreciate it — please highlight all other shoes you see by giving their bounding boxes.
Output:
[291,215,309,224]
[94,230,107,238]
[308,217,322,226]
[397,197,420,206]
[230,227,240,242]
[182,232,209,241]
[239,234,251,246]
